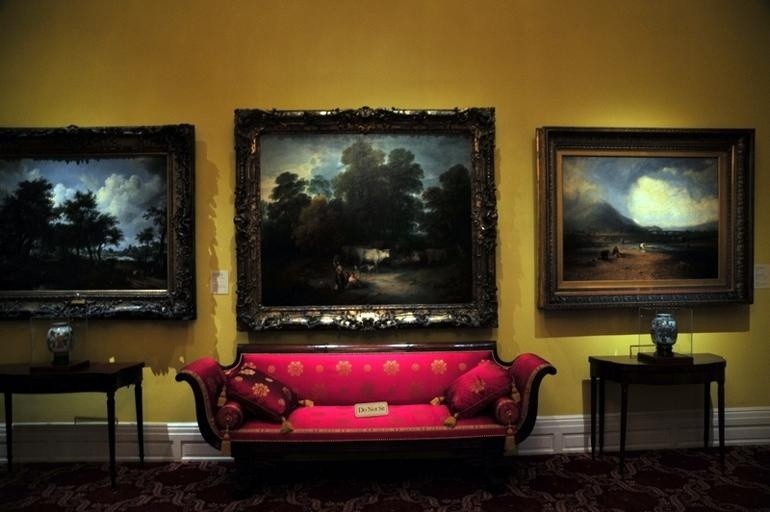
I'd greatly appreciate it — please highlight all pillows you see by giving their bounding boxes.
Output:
[429,359,521,428]
[216,367,314,435]
[494,396,522,433]
[215,396,244,457]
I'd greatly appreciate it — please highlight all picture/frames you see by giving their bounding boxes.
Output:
[233,106,500,332]
[0,123,196,321]
[534,125,751,314]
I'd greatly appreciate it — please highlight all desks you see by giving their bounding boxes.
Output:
[588,353,726,468]
[0,363,146,490]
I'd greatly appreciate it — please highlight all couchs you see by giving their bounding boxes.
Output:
[175,341,556,478]
